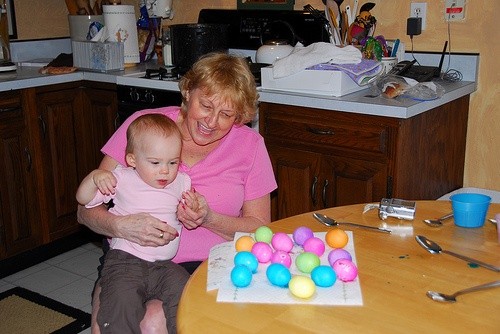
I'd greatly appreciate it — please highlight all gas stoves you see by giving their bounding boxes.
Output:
[116,9,329,106]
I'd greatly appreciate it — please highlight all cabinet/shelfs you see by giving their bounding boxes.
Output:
[0,79,120,262]
[258,102,400,223]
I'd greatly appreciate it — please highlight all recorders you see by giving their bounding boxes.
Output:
[199,10,327,86]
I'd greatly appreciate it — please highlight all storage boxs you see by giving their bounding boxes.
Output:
[261,68,375,94]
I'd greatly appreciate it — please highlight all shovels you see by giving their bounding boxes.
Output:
[304,4,332,35]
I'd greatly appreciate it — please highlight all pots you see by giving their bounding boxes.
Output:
[168,23,230,75]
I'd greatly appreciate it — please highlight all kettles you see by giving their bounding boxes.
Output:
[256,19,302,66]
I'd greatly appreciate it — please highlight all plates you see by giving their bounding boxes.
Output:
[401,95,439,101]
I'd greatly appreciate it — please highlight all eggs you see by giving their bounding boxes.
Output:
[231,264,251,288]
[293,226,313,246]
[295,252,320,273]
[303,237,325,257]
[234,251,258,272]
[288,275,315,299]
[271,250,291,268]
[328,249,352,267]
[325,229,348,248]
[271,233,293,252]
[254,225,273,244]
[333,258,358,282]
[235,236,255,252]
[266,262,291,287]
[311,265,336,287]
[251,241,273,263]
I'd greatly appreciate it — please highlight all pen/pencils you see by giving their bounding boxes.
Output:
[391,39,399,57]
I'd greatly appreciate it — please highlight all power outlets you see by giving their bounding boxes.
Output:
[410,2,427,31]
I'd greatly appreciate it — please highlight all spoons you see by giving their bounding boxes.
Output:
[423,212,453,227]
[426,279,500,303]
[414,234,500,272]
[313,212,392,234]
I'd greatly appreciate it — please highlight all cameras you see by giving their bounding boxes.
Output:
[379,198,417,221]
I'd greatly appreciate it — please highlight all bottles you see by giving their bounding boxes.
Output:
[163,37,173,67]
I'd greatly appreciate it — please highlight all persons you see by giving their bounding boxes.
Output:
[76,50,277,334]
[76,113,200,334]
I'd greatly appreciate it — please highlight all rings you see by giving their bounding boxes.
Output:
[159,230,164,238]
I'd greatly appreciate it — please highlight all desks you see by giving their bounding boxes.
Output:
[176,200,500,334]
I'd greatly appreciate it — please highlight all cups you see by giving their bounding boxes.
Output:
[381,57,398,73]
[67,14,103,42]
[450,193,492,228]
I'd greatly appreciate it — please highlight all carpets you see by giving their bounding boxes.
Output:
[0,286,92,334]
